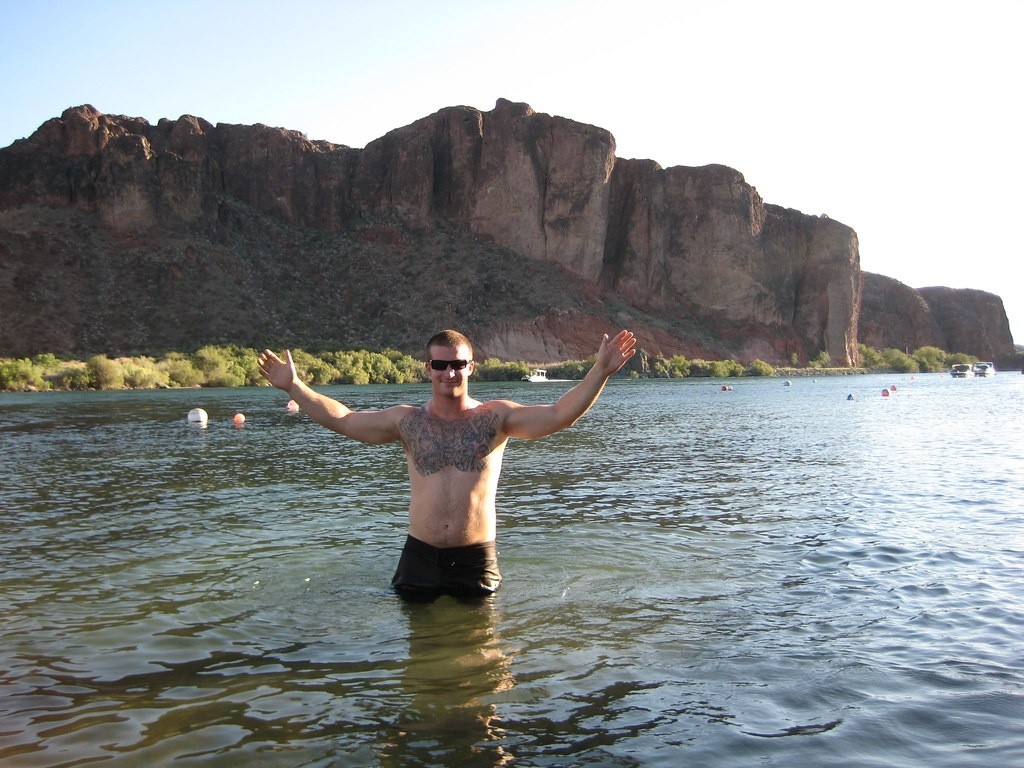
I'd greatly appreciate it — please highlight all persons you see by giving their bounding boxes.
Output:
[257,330,636,597]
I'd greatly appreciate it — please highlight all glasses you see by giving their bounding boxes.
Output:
[429,359,472,370]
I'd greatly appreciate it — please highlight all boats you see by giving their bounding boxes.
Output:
[949,363,970,379]
[973,362,995,378]
